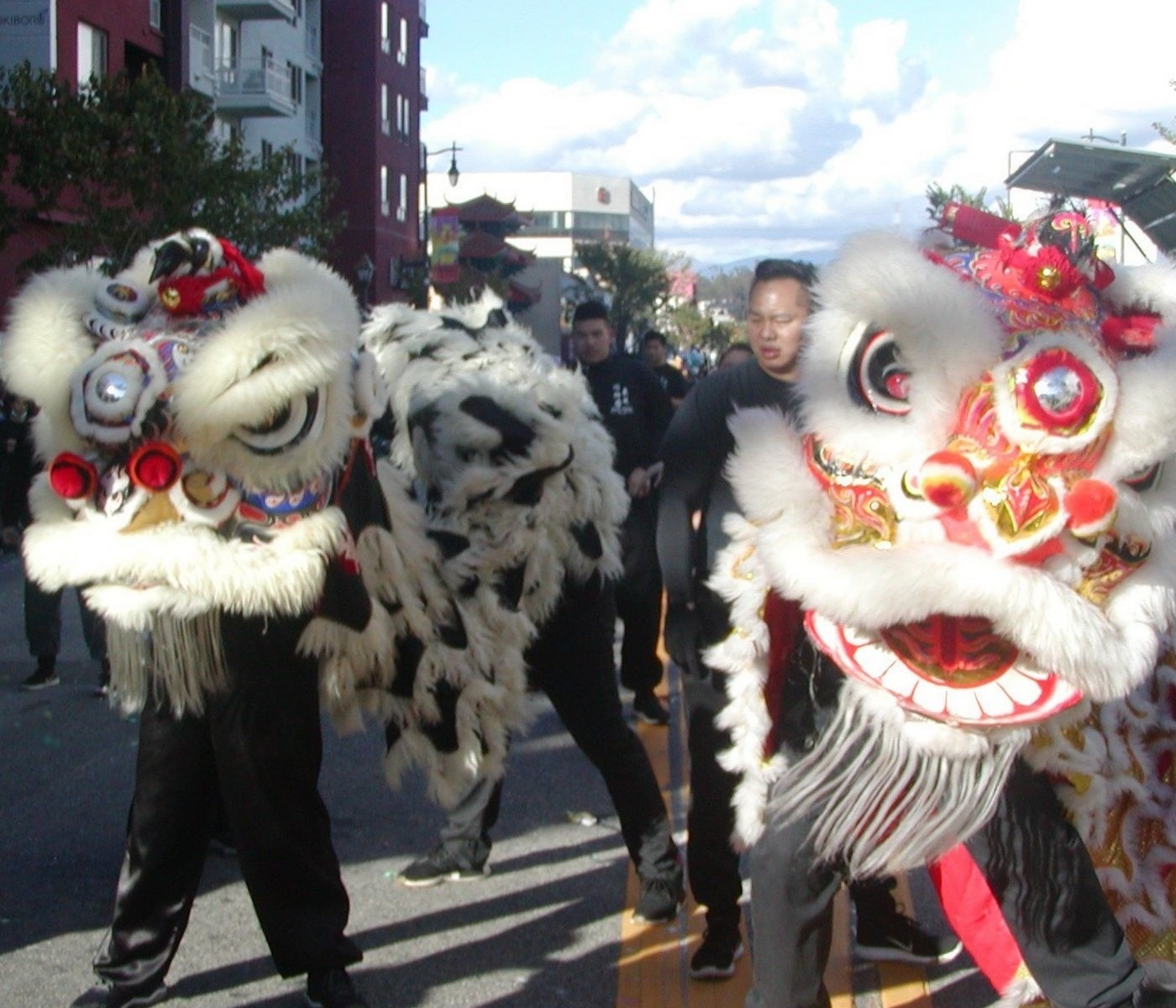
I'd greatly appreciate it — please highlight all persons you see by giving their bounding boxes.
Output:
[655,258,964,983]
[0,256,110,694]
[568,302,676,725]
[644,331,688,410]
[748,640,849,1007]
[71,611,363,1008]
[399,572,688,927]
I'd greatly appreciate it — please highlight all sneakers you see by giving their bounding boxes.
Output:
[396,843,490,887]
[69,977,166,1008]
[96,666,112,693]
[21,668,60,690]
[304,972,368,1008]
[853,902,963,965]
[632,694,670,726]
[690,927,743,978]
[631,856,687,925]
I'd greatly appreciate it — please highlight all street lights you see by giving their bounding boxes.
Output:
[421,141,464,309]
[1006,148,1039,214]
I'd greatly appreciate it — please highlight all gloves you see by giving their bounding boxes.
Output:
[664,606,708,678]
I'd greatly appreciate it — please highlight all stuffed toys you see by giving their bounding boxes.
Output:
[702,210,1176,1007]
[0,231,636,811]
